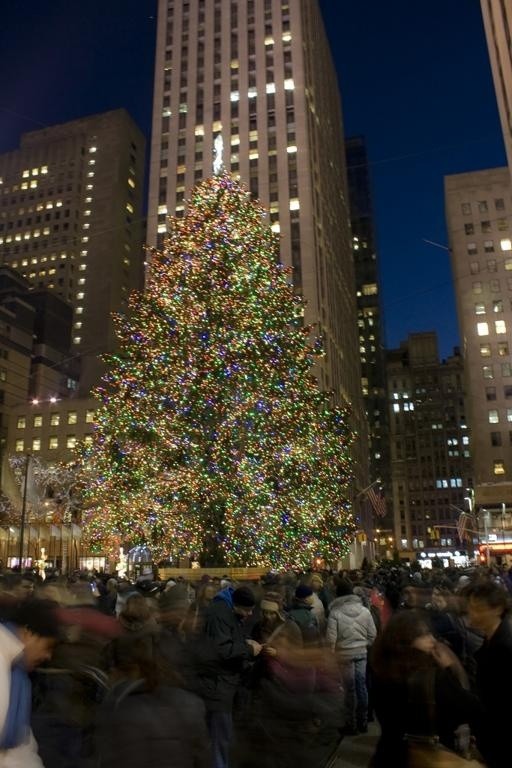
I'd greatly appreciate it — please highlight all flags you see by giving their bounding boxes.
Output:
[367,480,389,518]
[453,507,468,543]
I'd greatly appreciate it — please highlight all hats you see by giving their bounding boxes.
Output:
[335,582,353,596]
[260,591,288,624]
[296,584,313,600]
[231,586,254,608]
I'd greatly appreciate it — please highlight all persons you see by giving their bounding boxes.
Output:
[0,549,512,768]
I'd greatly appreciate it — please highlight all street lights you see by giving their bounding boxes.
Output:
[463,487,491,569]
